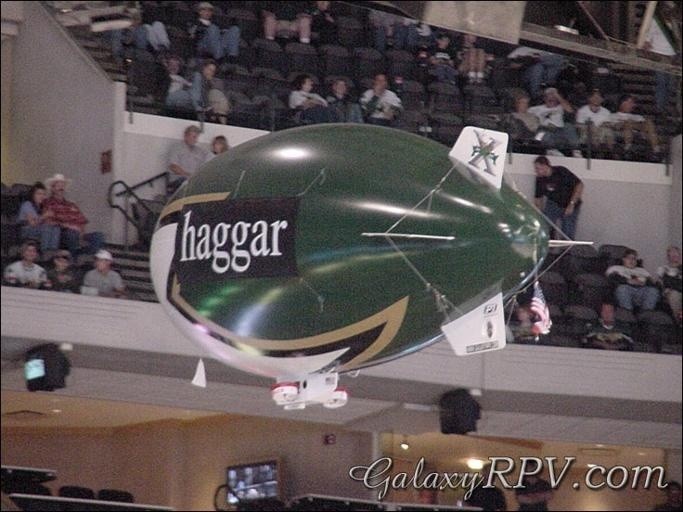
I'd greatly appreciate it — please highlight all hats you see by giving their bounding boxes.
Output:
[93,249,113,262]
[45,174,72,190]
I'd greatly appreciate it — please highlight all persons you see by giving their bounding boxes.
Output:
[504,245,683,354]
[514,456,553,512]
[532,156,585,242]
[71,1,666,159]
[462,463,507,512]
[3,240,47,289]
[228,463,278,505]
[42,247,82,293]
[168,125,210,188]
[641,1,682,127]
[420,470,442,505]
[205,134,232,162]
[14,182,61,250]
[41,174,102,254]
[653,481,683,510]
[83,249,126,298]
[26,475,53,504]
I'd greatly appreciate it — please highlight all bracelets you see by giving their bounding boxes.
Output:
[568,200,576,206]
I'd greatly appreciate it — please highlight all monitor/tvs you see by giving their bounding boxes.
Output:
[439,390,481,433]
[225,457,282,505]
[22,345,70,392]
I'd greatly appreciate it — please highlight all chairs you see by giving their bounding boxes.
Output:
[1,182,121,297]
[502,243,683,355]
[41,1,660,164]
